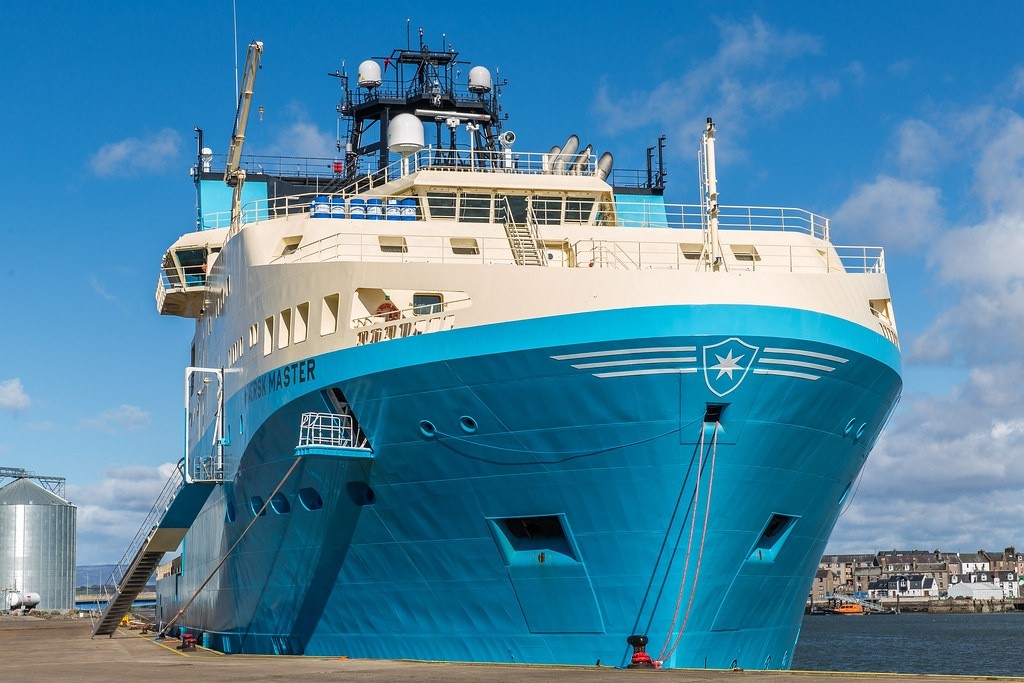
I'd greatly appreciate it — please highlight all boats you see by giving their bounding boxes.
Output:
[154,0,904,671]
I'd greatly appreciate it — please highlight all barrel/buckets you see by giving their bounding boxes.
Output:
[366,198,382,220]
[387,199,401,220]
[401,199,416,221]
[310,198,314,218]
[314,196,330,218]
[350,199,364,219]
[331,197,345,218]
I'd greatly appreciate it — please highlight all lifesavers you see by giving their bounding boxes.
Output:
[633,653,649,661]
[377,302,399,321]
